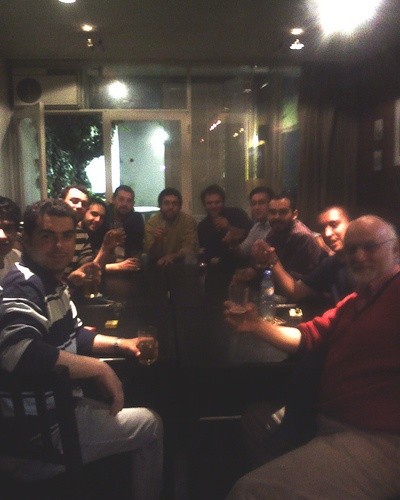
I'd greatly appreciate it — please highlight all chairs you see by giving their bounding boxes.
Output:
[0,364,87,500]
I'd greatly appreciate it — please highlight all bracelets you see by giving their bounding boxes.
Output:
[269,254,279,265]
[112,337,121,353]
[65,278,84,291]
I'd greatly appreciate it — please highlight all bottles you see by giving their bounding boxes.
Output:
[260,270,276,321]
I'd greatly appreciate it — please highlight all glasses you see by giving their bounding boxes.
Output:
[343,238,398,252]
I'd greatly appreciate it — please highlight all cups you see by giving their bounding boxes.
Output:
[113,242,125,263]
[227,283,250,322]
[83,268,98,299]
[138,326,158,365]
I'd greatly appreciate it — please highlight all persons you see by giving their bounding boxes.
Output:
[220,215,400,500]
[0,183,351,313]
[0,198,164,500]
